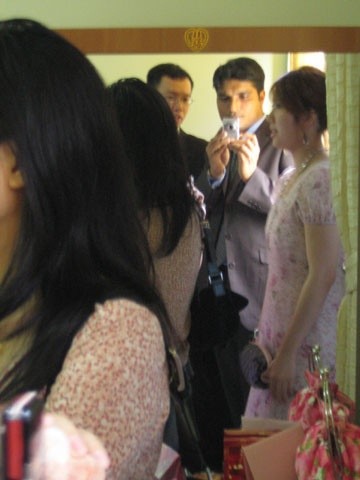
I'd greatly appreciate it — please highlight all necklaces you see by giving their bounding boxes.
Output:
[280,146,327,199]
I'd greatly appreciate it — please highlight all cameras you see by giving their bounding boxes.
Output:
[222,117,239,141]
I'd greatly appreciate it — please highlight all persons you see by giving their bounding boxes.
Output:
[242,65,349,423]
[188,56,295,472]
[146,63,211,186]
[106,74,210,456]
[0,16,171,480]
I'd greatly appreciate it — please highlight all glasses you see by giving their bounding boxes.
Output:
[164,94,193,105]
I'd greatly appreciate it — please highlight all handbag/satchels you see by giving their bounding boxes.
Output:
[188,271,250,351]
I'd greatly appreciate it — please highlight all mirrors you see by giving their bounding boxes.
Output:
[54,25,360,480]
